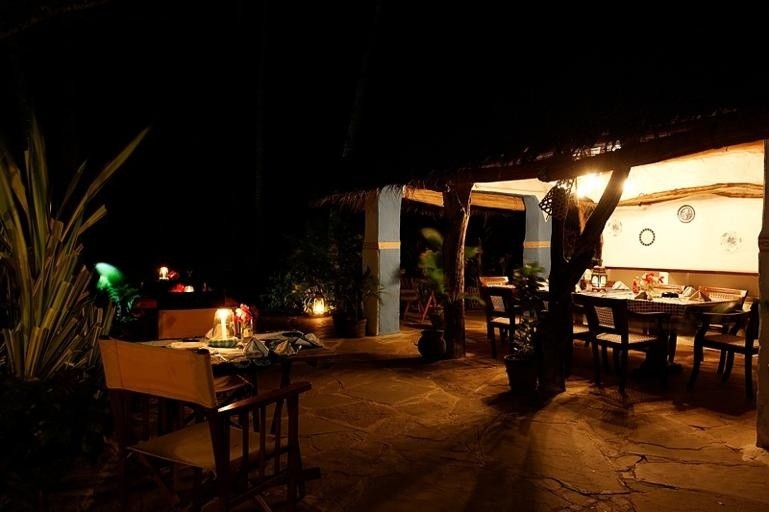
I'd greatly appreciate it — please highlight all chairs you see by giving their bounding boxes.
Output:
[476,275,759,400]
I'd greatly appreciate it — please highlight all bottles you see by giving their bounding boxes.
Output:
[236,302,254,344]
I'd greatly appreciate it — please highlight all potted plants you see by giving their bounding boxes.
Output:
[332,270,384,338]
[419,227,485,330]
[504,262,548,392]
[259,250,335,339]
